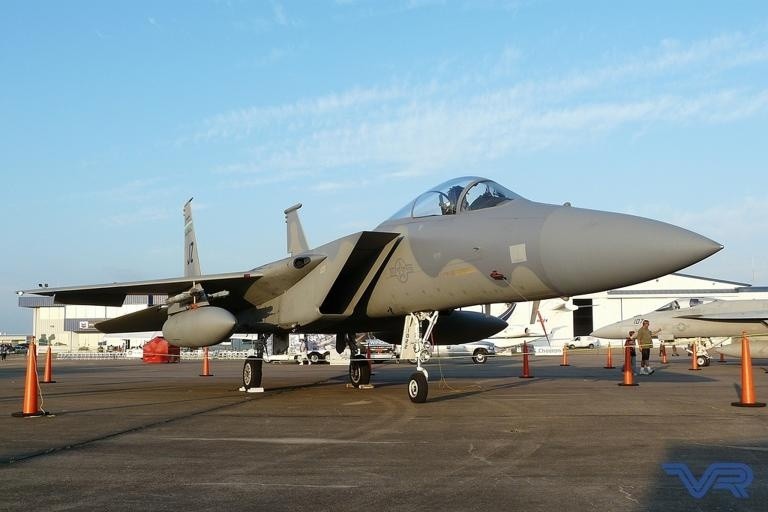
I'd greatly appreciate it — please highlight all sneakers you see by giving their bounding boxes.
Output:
[639,371,648,376]
[648,369,654,376]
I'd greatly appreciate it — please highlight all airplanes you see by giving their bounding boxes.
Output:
[14,176,726,404]
[588,296,768,367]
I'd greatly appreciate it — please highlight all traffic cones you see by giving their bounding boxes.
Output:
[43,342,52,383]
[521,338,531,378]
[561,340,726,386]
[202,346,210,377]
[739,330,757,406]
[22,334,38,416]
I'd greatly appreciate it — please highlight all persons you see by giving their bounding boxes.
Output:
[299,338,312,367]
[622,330,638,377]
[637,319,662,376]
[447,185,470,211]
[0,342,8,364]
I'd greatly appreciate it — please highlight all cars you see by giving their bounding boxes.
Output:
[13,344,28,353]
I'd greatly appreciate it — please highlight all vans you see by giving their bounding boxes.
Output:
[392,341,496,364]
[565,335,601,349]
